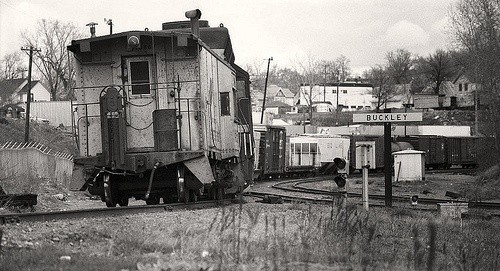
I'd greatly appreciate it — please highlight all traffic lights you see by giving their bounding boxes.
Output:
[333,157,347,188]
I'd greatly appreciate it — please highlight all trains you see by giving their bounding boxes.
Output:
[67,8,496,208]
